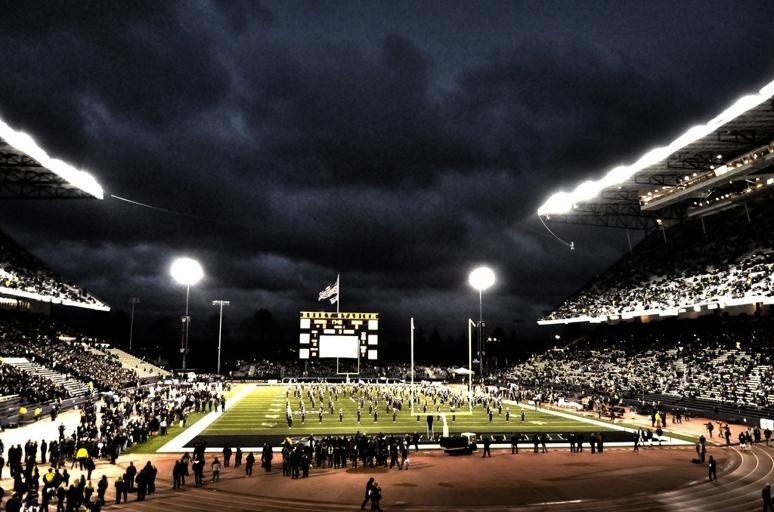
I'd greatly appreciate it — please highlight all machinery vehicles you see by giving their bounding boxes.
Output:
[438,431,478,456]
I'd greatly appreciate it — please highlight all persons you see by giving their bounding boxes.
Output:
[361,478,383,512]
[1,232,274,512]
[467,198,773,512]
[276,363,467,480]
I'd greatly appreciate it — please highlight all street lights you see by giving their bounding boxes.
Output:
[210,298,230,373]
[168,256,205,372]
[468,264,497,379]
[485,336,500,369]
[127,295,141,349]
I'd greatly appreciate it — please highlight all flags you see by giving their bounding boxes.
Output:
[317,276,338,301]
[329,294,337,305]
